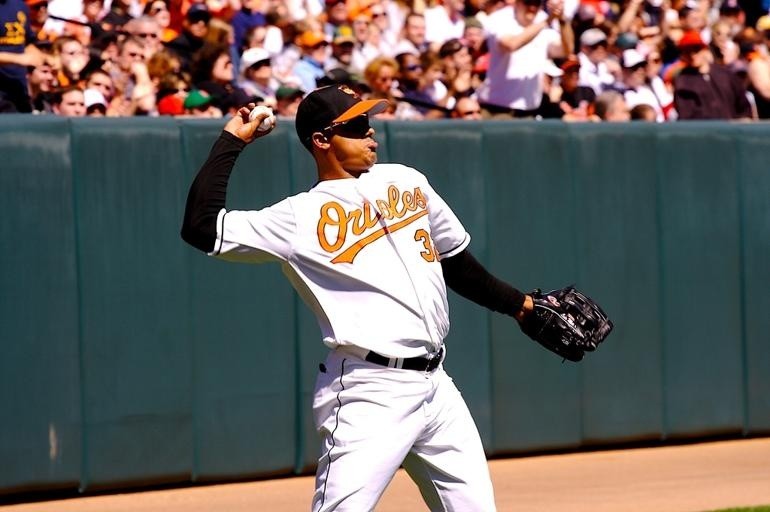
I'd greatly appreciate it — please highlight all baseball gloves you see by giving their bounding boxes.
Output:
[520,285,613,362]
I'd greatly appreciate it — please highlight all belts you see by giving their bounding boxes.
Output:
[365,347,447,374]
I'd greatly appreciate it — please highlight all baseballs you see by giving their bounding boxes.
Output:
[249,105,274,132]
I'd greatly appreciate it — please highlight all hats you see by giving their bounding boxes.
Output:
[545,30,709,78]
[294,82,390,140]
[82,30,304,113]
[298,27,491,84]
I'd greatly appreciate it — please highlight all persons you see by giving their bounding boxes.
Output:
[178,83,613,512]
